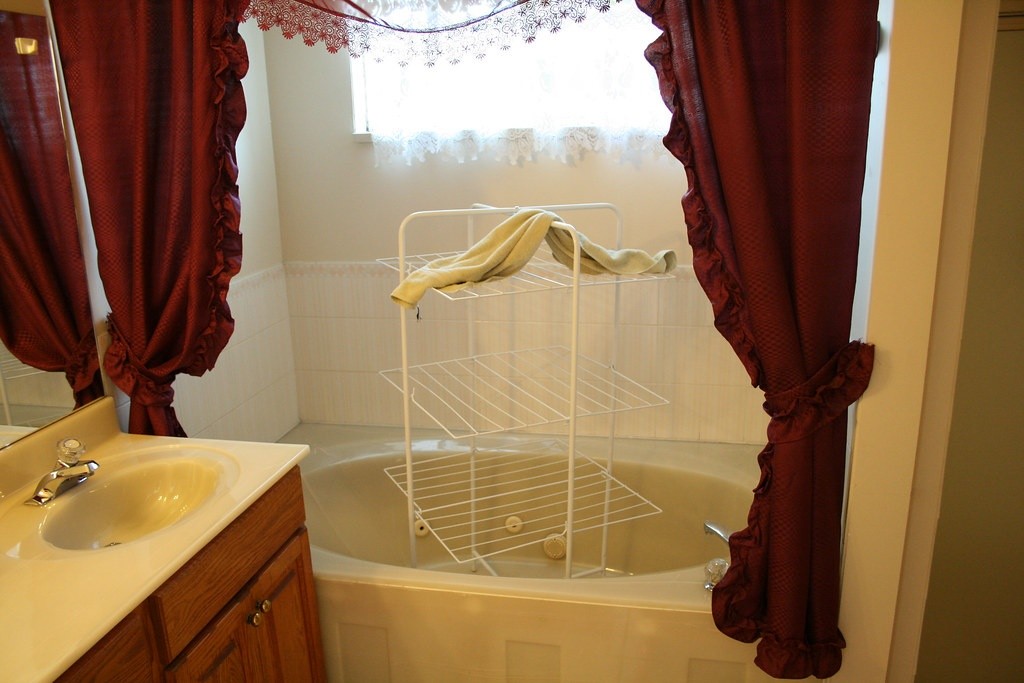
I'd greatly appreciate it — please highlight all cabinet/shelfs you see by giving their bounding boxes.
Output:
[375,205,675,578]
[53,465,327,682]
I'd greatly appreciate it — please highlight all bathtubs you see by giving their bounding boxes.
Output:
[226,422,826,683]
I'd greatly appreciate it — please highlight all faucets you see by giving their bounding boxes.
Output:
[22,458,100,507]
[703,519,736,545]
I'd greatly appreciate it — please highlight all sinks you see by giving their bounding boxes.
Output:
[38,443,241,554]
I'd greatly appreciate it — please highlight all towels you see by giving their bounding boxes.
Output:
[388,207,680,310]
